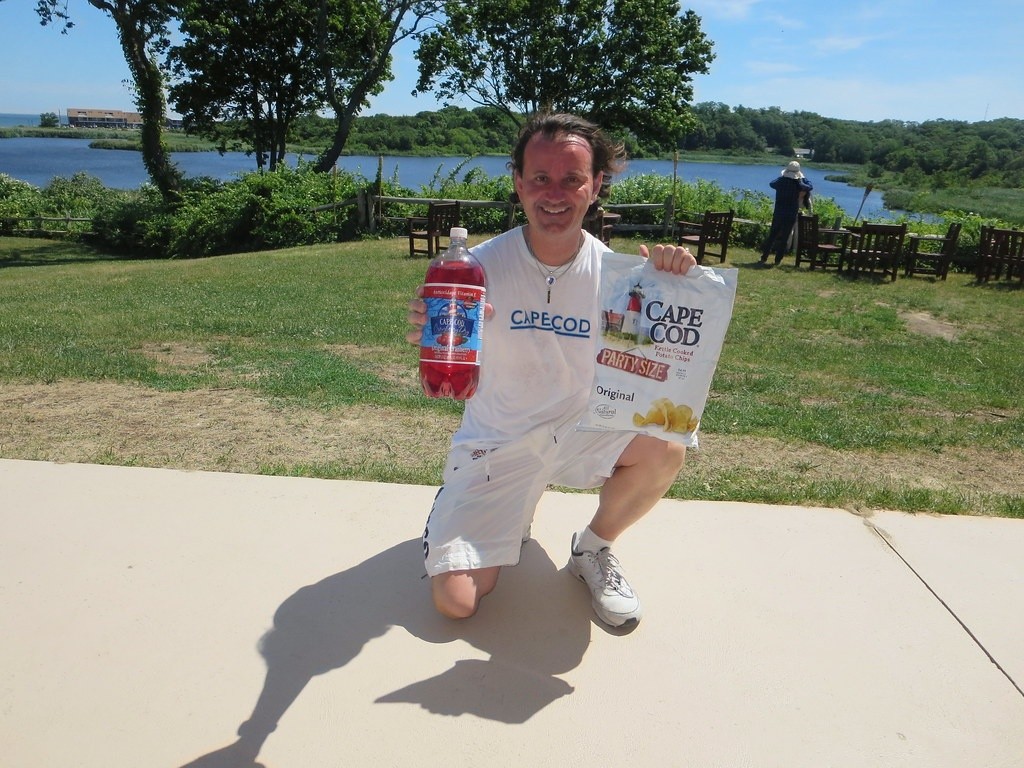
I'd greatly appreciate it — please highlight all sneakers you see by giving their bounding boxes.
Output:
[523,525,531,541]
[566,531,641,629]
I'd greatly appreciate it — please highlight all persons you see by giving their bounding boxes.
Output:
[758,160,813,266]
[406,111,696,630]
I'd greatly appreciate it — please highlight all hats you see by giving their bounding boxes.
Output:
[780,161,804,179]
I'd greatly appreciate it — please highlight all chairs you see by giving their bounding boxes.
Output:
[583,207,621,251]
[975,224,1024,283]
[844,220,907,282]
[794,211,852,273]
[409,200,461,259]
[678,209,734,266]
[904,222,961,281]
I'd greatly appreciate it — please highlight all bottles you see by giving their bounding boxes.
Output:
[420,227,486,402]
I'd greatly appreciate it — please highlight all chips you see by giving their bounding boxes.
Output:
[633,398,699,434]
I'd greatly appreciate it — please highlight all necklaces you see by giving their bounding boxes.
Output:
[527,223,585,303]
